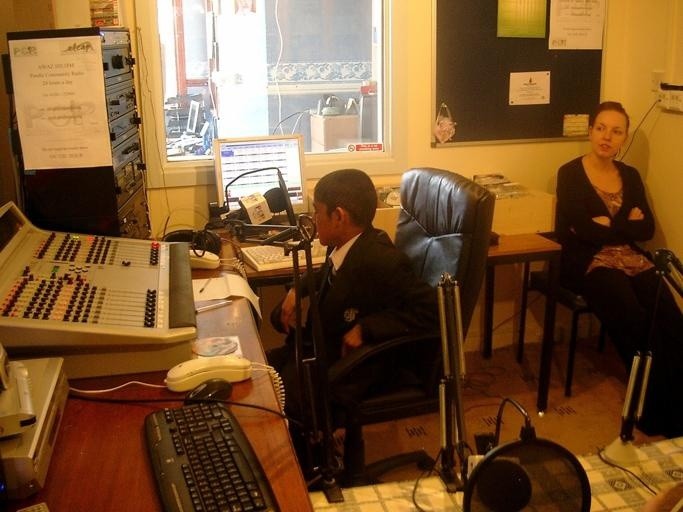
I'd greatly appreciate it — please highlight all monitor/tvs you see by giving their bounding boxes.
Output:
[213,134,308,221]
[185,99,200,137]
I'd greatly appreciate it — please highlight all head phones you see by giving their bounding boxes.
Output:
[159,226,222,255]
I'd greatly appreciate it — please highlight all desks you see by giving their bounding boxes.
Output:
[231,231,566,411]
[0,226,317,512]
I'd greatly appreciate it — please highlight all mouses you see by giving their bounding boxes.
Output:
[182,377,234,406]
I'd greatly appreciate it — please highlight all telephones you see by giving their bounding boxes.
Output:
[200,278,211,292]
[189,249,220,270]
[166,355,253,393]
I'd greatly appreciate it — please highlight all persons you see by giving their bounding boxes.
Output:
[264,169,436,490]
[551,102,683,440]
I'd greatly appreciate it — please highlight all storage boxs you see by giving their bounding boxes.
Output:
[308,111,363,154]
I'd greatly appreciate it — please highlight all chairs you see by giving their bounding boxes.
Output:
[514,188,609,414]
[270,166,497,488]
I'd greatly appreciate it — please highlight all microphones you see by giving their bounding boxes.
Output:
[475,459,532,512]
[225,187,287,229]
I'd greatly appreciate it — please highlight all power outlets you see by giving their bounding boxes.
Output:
[655,82,683,114]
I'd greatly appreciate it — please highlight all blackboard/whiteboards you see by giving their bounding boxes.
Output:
[431,0,609,147]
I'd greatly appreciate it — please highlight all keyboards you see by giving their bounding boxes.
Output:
[142,400,285,512]
[240,238,329,272]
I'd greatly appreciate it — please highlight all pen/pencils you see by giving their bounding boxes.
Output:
[197,300,232,313]
